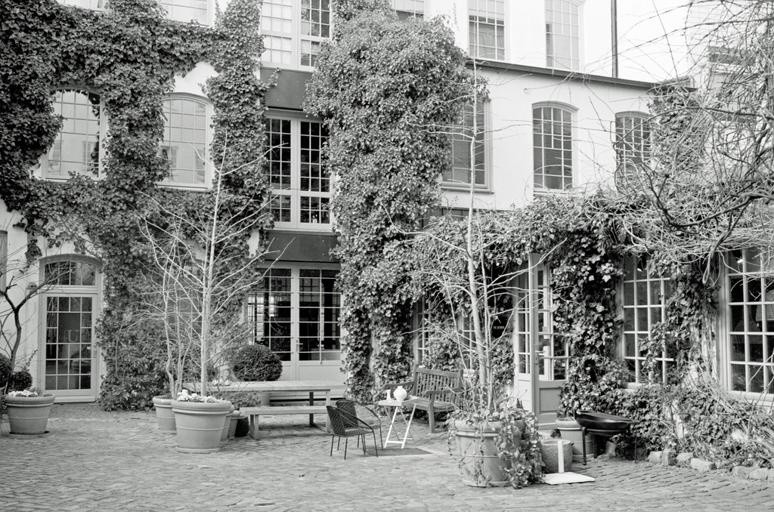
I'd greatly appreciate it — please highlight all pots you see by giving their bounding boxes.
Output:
[576,411,626,432]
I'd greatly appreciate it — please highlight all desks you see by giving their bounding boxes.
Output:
[182,375,333,431]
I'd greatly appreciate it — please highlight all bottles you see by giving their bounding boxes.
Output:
[386,390,392,402]
[393,386,407,402]
[210,374,231,387]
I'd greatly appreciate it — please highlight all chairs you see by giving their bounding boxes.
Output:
[325,365,466,459]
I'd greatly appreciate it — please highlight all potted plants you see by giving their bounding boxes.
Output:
[392,52,560,486]
[541,407,594,473]
[4,387,57,436]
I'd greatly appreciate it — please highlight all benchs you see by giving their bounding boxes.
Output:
[240,404,326,441]
